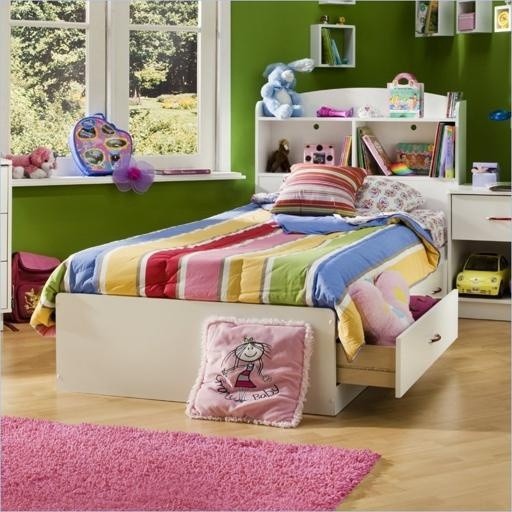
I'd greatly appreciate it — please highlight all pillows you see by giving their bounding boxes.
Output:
[270,163,423,218]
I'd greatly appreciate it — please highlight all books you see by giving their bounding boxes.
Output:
[446,92,464,119]
[339,121,455,180]
[152,168,211,175]
[321,27,348,66]
[489,182,510,192]
[424,1,438,35]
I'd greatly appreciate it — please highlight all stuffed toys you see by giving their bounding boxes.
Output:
[4,146,59,179]
[260,59,316,120]
[265,139,291,172]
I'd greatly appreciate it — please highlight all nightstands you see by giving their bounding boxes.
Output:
[447,179,512,322]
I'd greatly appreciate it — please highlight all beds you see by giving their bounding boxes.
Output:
[53,209,459,416]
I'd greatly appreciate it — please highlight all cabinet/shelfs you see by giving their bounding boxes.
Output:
[255,86,467,209]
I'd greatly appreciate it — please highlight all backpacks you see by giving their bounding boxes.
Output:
[3,249,62,334]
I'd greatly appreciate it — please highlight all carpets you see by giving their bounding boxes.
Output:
[0,415,382,510]
